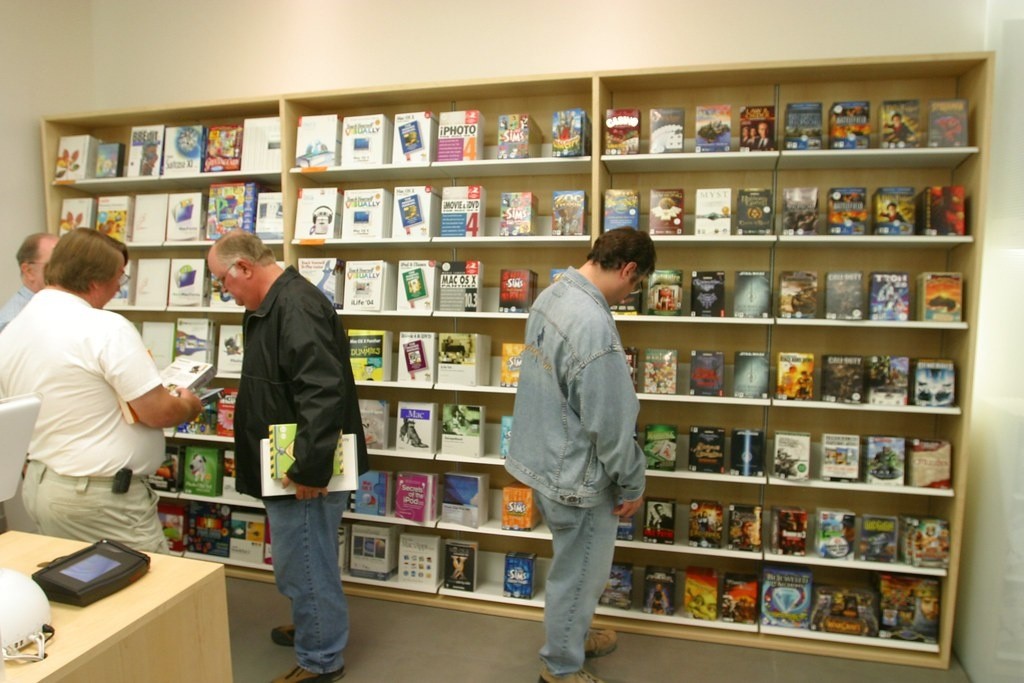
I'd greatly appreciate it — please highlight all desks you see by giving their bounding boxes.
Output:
[0,530,231,683]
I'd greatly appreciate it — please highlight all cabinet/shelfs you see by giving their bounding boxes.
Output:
[42,53,993,671]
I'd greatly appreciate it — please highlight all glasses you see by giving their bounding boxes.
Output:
[212,261,239,287]
[114,265,134,285]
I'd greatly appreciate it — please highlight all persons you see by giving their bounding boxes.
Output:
[0,234,62,534]
[174,387,239,437]
[504,226,657,683]
[0,227,204,556]
[207,230,370,683]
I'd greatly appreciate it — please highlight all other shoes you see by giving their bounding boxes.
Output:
[537,663,606,683]
[270,659,347,683]
[271,625,295,647]
[584,629,619,659]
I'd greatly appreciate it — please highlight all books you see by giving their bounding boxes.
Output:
[296,108,591,171]
[603,185,967,235]
[345,329,526,388]
[259,424,359,497]
[48,182,284,245]
[145,446,259,501]
[293,186,587,238]
[298,257,568,315]
[597,269,963,646]
[336,521,537,600]
[157,496,273,565]
[357,399,515,459]
[131,318,244,373]
[102,258,285,312]
[161,355,216,397]
[604,99,969,156]
[51,116,283,180]
[354,469,543,531]
